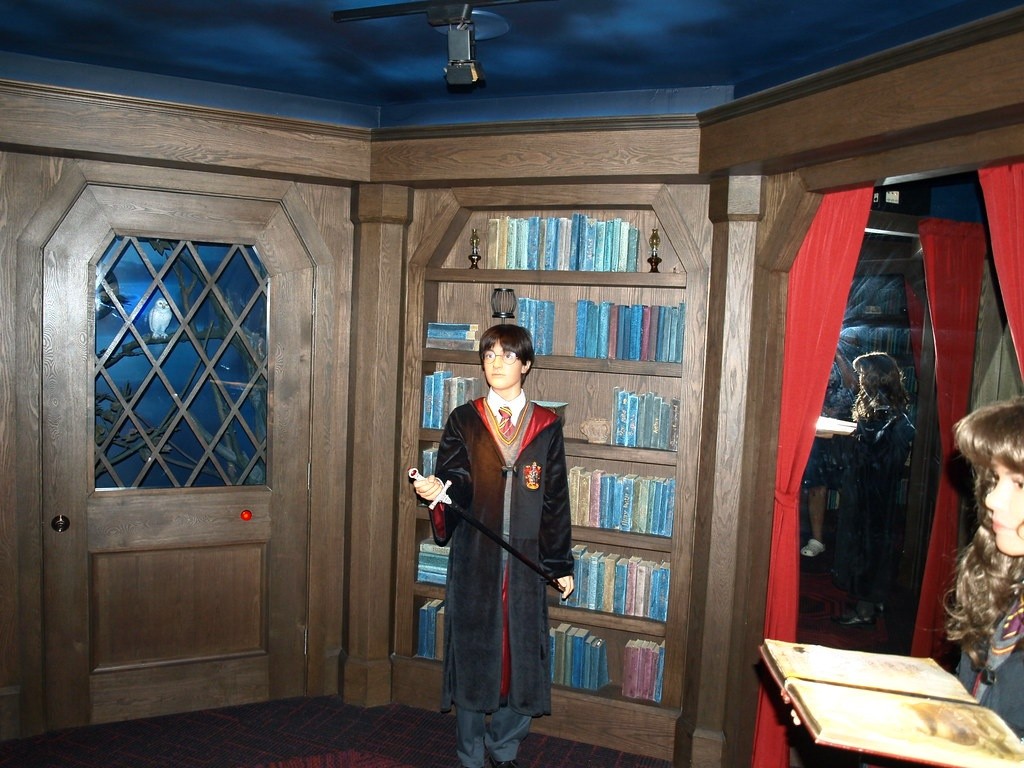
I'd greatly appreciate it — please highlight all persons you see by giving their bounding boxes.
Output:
[802,326,869,557]
[836,353,922,629]
[414,324,575,768]
[790,395,1024,744]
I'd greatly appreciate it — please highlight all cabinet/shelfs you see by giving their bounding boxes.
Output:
[393,184,729,758]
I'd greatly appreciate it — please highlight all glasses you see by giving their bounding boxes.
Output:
[482,349,518,365]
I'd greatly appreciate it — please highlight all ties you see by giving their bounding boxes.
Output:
[498,406,516,442]
[1000,590,1024,639]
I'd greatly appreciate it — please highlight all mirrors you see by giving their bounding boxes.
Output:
[795,212,979,648]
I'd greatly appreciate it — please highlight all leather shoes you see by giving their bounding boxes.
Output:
[834,604,887,629]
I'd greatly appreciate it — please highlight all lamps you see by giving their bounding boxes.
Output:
[445,18,480,86]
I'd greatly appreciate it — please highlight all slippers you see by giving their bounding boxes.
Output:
[801,539,826,558]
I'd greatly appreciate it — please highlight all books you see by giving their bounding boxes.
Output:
[487,212,639,272]
[611,386,679,452]
[417,599,445,661]
[426,322,480,352]
[423,441,440,478]
[574,299,686,363]
[560,544,671,622]
[549,623,609,691]
[417,537,452,586]
[621,638,665,703]
[516,297,555,357]
[802,276,918,510]
[758,638,1024,768]
[422,370,480,430]
[567,466,676,537]
